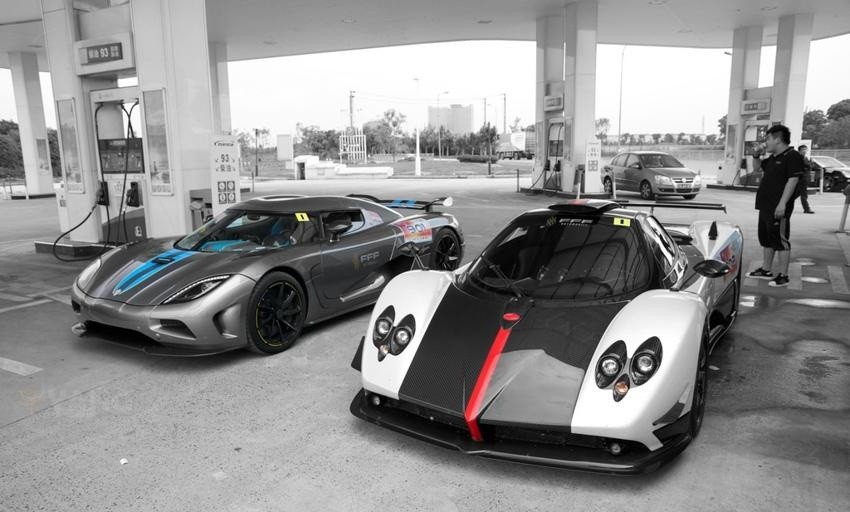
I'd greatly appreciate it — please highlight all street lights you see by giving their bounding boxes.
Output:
[255,128,260,178]
[486,119,493,175]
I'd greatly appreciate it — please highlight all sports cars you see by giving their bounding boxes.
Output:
[69,193,466,361]
[350,193,747,472]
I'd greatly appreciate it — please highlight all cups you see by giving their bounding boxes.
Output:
[753,143,768,152]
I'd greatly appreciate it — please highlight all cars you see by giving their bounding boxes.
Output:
[602,150,703,201]
[806,154,850,192]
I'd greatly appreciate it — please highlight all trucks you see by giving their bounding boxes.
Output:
[495,132,535,160]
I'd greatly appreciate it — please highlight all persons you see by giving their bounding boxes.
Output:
[745,124,803,287]
[799,146,817,215]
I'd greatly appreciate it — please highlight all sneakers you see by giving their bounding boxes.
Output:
[745,268,774,280]
[768,272,790,288]
